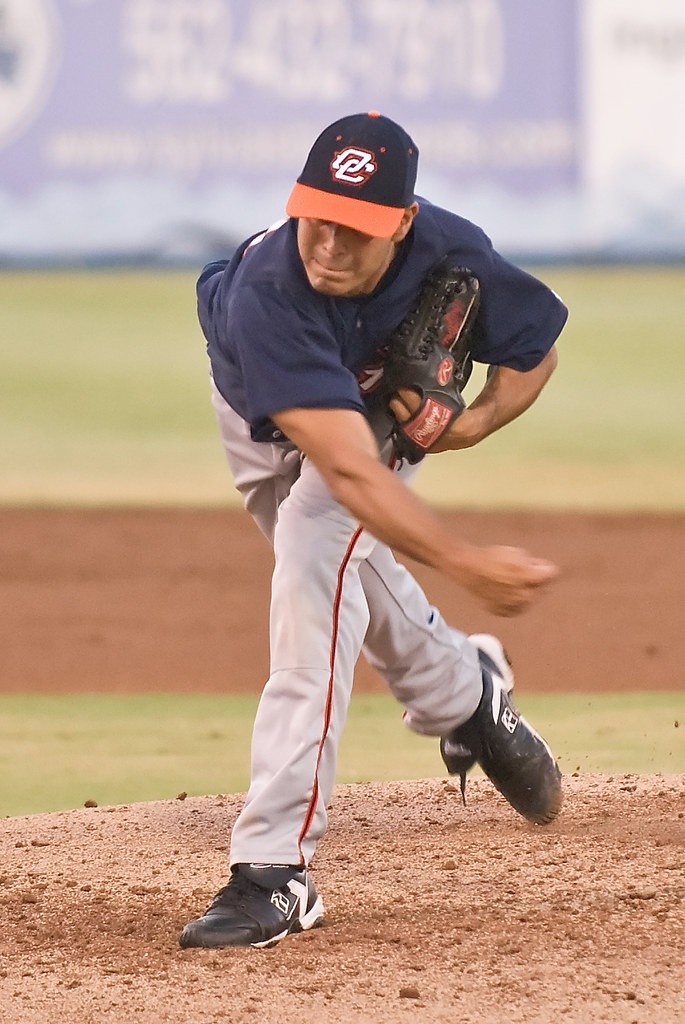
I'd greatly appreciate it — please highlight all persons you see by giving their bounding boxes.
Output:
[177,110,570,952]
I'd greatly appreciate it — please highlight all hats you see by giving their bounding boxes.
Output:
[288,112,417,242]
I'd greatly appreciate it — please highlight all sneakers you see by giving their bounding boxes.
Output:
[178,863,325,951]
[438,633,563,829]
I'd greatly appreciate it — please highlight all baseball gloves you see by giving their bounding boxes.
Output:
[379,264,480,464]
